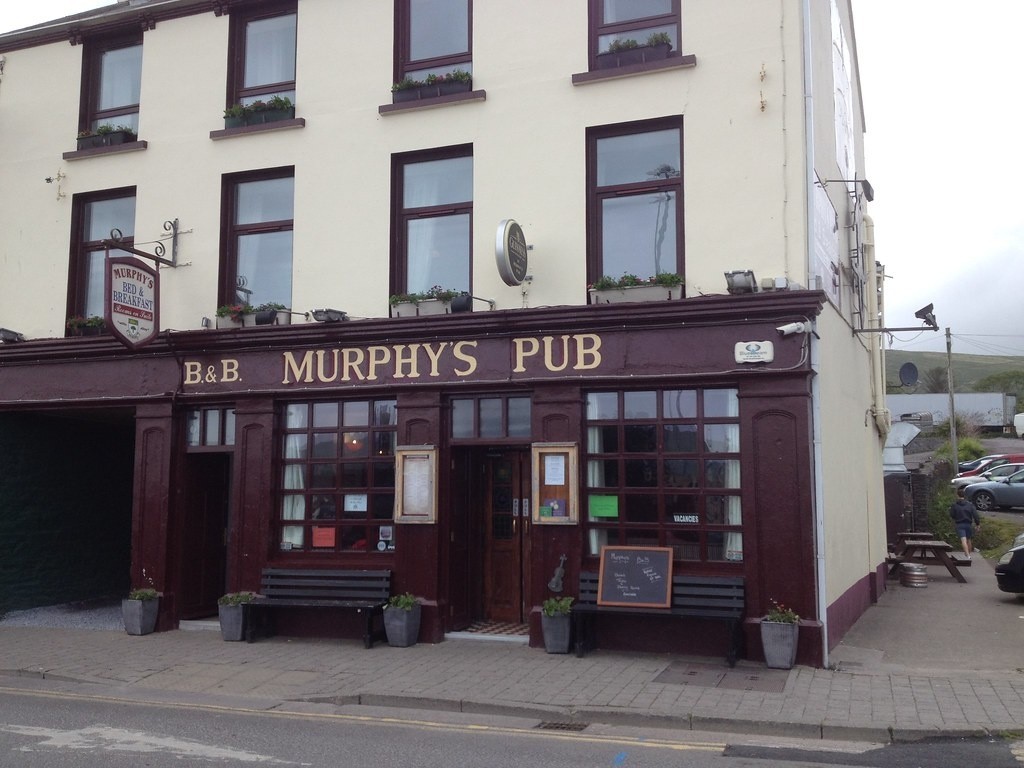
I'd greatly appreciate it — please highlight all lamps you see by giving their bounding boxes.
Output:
[824,179,874,202]
[723,269,759,295]
[851,304,940,334]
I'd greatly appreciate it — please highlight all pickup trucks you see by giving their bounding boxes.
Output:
[1012,412,1024,440]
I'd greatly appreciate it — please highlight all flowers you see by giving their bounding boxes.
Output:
[217,302,244,321]
[766,597,801,624]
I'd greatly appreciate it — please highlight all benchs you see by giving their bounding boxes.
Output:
[569,572,746,666]
[242,567,392,649]
[887,552,972,567]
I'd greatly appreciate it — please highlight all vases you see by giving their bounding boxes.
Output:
[215,316,244,330]
[760,615,798,670]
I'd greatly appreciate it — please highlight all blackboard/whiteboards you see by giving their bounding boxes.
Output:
[597,545,673,607]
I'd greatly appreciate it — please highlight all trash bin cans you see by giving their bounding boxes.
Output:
[901,412,933,434]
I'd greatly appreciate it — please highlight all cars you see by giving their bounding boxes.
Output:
[995,543,1024,594]
[951,462,1024,497]
[963,468,1024,511]
[959,454,1006,471]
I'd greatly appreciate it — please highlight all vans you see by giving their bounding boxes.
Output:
[956,454,1024,477]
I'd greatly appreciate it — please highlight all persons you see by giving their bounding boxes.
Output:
[950,491,980,559]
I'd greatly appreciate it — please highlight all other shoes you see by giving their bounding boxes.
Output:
[967,556,972,560]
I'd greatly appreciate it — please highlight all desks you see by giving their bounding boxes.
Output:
[888,540,967,583]
[891,532,934,555]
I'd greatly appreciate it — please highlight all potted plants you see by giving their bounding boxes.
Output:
[121,589,158,635]
[218,593,251,641]
[68,31,683,337]
[382,593,420,647]
[541,596,574,653]
